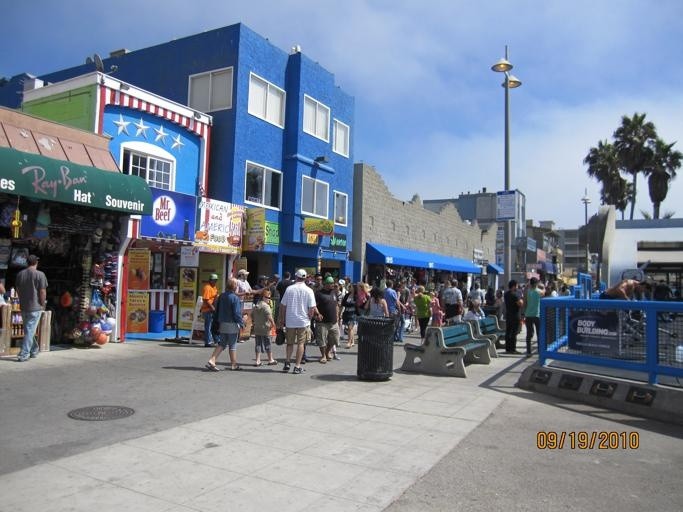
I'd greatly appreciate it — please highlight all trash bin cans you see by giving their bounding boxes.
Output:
[355,315,395,382]
[150,311,164,333]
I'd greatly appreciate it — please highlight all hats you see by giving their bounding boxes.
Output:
[238,269,249,275]
[27,255,40,260]
[257,274,270,280]
[210,273,219,280]
[295,269,307,278]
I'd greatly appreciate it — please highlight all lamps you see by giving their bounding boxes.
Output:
[317,157,329,164]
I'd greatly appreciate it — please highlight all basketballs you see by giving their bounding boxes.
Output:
[90,326,101,339]
[96,333,108,345]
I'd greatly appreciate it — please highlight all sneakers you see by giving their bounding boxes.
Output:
[18,346,39,362]
[205,343,217,346]
[319,355,341,363]
[506,349,520,354]
[283,358,309,374]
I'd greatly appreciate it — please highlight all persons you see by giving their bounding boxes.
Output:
[197,268,680,376]
[14,251,48,363]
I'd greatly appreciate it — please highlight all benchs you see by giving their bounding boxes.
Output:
[400,314,507,379]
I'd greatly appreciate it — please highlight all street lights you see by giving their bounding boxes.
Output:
[580,187,591,226]
[490,44,522,191]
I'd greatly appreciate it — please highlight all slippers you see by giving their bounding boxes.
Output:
[206,364,219,371]
[231,365,242,370]
[255,359,279,367]
